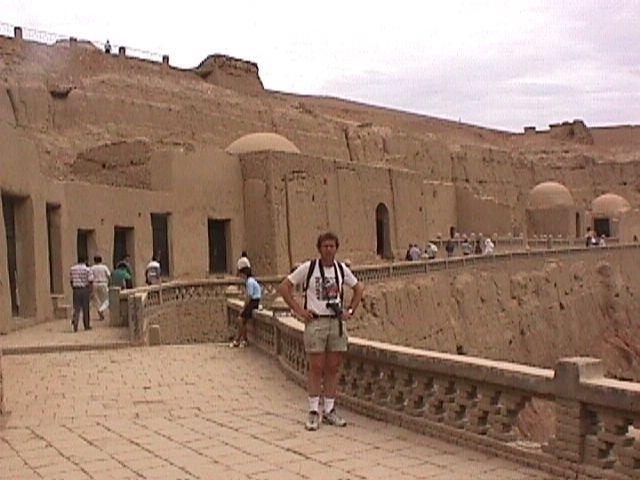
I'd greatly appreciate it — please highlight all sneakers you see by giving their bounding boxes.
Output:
[322,409,346,427]
[230,339,249,348]
[306,411,322,431]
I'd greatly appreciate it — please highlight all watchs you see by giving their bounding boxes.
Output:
[346,307,355,316]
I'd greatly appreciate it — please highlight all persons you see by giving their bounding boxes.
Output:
[403,236,494,262]
[104,38,112,54]
[67,251,163,333]
[236,249,252,277]
[278,231,367,433]
[227,266,262,350]
[583,226,608,250]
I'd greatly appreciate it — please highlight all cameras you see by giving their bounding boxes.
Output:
[326,302,343,315]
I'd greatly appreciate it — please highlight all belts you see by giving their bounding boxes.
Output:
[312,314,337,318]
